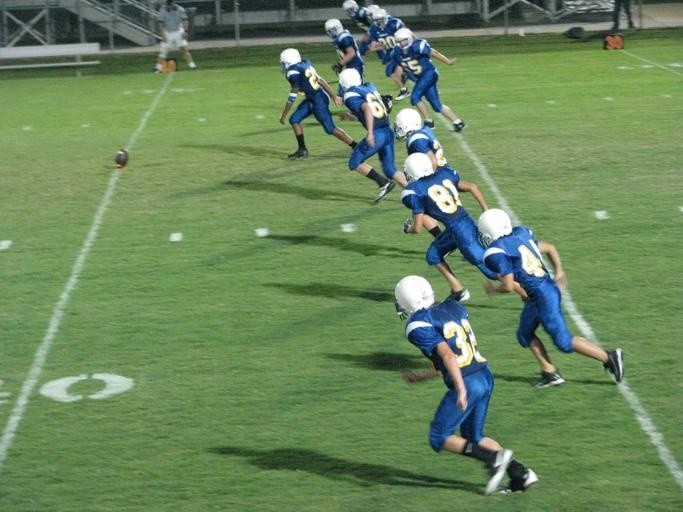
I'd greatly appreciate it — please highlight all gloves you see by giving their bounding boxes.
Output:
[330,62,343,75]
[400,216,412,235]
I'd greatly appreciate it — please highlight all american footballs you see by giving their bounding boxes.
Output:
[116,149,128,168]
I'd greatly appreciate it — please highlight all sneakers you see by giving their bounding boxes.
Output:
[444,288,471,305]
[372,180,396,204]
[481,446,514,498]
[603,347,627,383]
[393,88,411,101]
[496,466,540,498]
[286,148,309,161]
[423,119,435,131]
[452,121,465,133]
[400,72,409,84]
[530,370,566,390]
[380,94,393,114]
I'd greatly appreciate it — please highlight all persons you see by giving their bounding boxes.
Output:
[393,108,625,387]
[153,0,197,74]
[279,1,465,203]
[395,274,539,497]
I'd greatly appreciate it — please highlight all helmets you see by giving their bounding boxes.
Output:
[393,273,436,314]
[402,151,435,183]
[393,27,414,46]
[323,18,344,37]
[336,67,364,92]
[341,0,359,13]
[393,108,424,138]
[476,207,514,243]
[366,4,381,17]
[371,8,389,25]
[278,48,302,69]
